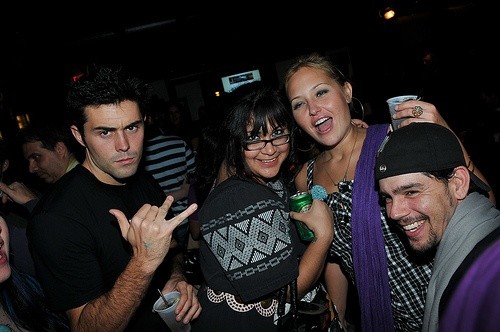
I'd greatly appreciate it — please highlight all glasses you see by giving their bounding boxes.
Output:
[240,130,291,151]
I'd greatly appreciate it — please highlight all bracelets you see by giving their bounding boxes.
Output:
[468,156,471,168]
[471,162,475,172]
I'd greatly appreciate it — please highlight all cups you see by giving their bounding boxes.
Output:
[153,292,191,332]
[386,95,418,130]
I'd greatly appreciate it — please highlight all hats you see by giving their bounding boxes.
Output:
[374,122,491,193]
[20,119,65,147]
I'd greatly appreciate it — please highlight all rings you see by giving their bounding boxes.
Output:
[412,106,423,118]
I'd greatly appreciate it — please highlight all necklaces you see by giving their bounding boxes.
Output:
[323,128,358,186]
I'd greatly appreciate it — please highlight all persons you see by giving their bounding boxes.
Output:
[0,52,500,332]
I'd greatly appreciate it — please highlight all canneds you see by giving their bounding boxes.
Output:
[289,190,315,242]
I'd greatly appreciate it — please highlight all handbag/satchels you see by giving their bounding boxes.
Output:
[182,251,204,279]
[277,279,344,332]
